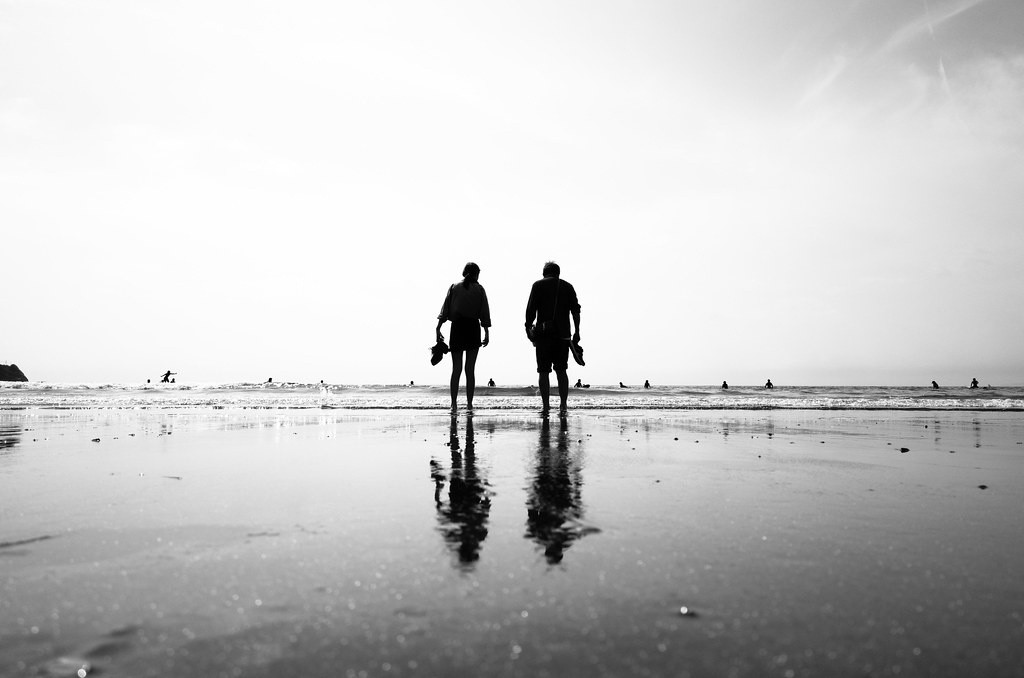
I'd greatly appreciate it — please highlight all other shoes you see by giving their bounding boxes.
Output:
[431,341,449,366]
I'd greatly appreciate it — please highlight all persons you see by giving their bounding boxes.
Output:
[147,364,980,390]
[526,414,602,565]
[430,415,490,563]
[436,262,491,413]
[525,262,582,413]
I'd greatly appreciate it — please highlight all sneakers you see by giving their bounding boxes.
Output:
[569,340,585,366]
